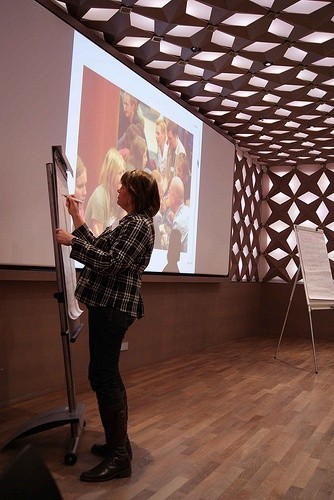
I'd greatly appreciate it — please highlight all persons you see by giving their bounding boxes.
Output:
[149,170,168,230]
[174,152,191,207]
[166,119,186,170]
[56,169,160,482]
[167,176,190,252]
[154,116,168,172]
[124,137,150,173]
[75,156,89,217]
[117,93,146,149]
[84,148,128,237]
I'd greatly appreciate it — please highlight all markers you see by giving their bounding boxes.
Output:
[61,193,83,204]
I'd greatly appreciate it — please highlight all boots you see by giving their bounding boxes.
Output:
[92,393,132,457]
[81,404,131,482]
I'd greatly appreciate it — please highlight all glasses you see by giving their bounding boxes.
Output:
[130,168,136,177]
